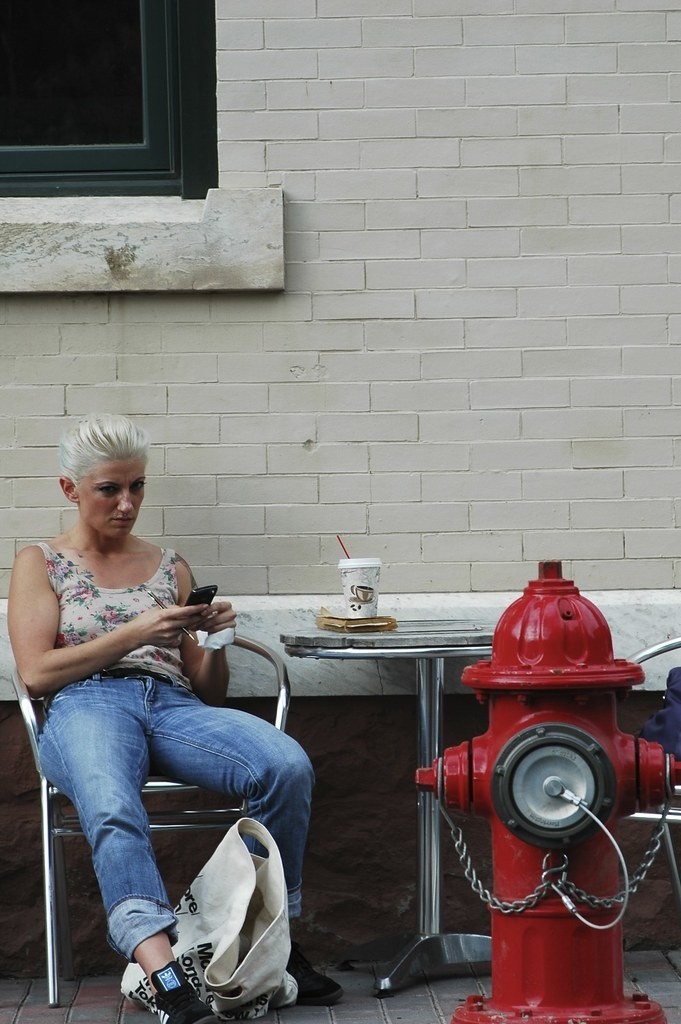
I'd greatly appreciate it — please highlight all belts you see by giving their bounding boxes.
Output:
[43,667,191,710]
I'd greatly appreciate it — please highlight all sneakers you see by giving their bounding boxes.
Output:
[151,960,219,1024]
[286,941,344,1005]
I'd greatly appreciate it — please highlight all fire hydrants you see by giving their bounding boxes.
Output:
[414,559,681,1024]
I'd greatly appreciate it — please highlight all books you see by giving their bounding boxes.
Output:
[316,607,399,633]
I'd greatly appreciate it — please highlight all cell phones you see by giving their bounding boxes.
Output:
[185,585,218,616]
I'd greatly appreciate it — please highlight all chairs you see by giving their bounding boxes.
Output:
[624,637,681,918]
[11,635,291,1007]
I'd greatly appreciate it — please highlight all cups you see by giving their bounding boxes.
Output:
[338,557,384,618]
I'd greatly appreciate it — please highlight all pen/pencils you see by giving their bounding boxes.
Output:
[147,589,197,642]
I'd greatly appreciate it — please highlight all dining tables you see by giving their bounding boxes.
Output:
[282,622,495,997]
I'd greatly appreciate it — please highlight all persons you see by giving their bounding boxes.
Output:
[7,416,345,1024]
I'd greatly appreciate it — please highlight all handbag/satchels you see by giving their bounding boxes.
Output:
[120,817,299,1023]
